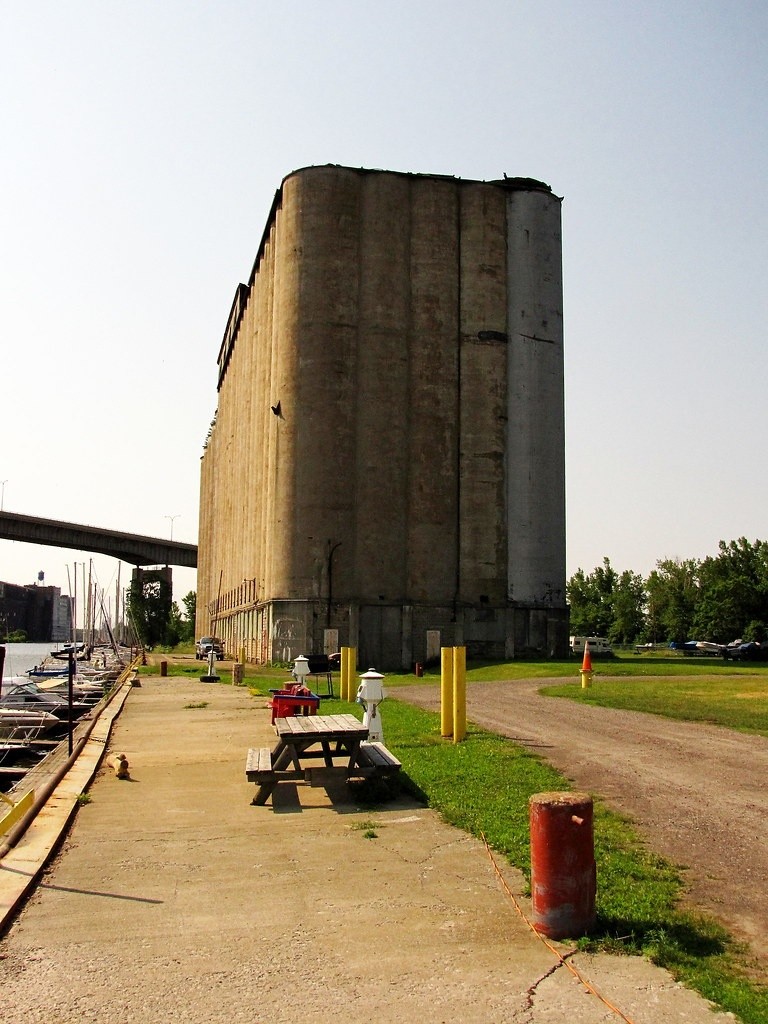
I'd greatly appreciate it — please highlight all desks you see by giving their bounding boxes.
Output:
[287,668,334,698]
[250,714,384,805]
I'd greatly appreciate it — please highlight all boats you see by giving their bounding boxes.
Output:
[634,642,666,653]
[695,639,743,654]
[669,640,706,656]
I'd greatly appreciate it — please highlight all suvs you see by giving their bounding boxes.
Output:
[195,636,225,661]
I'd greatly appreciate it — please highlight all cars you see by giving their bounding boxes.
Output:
[720,641,760,662]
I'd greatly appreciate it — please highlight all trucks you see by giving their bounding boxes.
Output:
[569,634,613,655]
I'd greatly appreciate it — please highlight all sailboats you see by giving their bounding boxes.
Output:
[0,556,139,779]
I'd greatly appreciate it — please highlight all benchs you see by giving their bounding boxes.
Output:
[244,748,272,782]
[361,741,402,769]
[269,685,320,724]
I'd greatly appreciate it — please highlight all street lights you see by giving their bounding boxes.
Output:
[164,514,182,541]
[0,480,8,511]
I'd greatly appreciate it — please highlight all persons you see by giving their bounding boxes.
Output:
[94,652,106,667]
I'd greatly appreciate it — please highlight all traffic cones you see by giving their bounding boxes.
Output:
[578,641,594,671]
